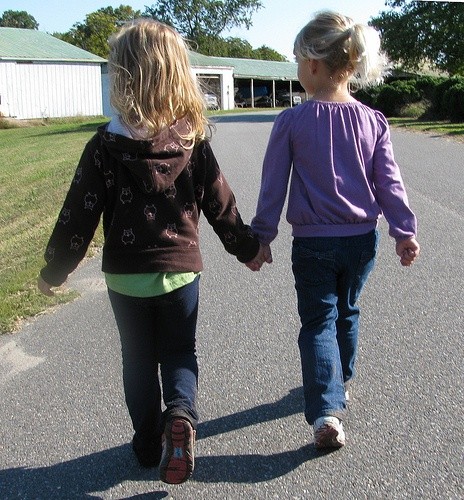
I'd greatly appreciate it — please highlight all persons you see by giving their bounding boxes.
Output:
[38,18,271,484]
[249,11,420,451]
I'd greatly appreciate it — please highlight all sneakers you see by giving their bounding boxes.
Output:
[344,390,350,401]
[314,416,345,447]
[159,417,196,485]
[132,432,163,468]
